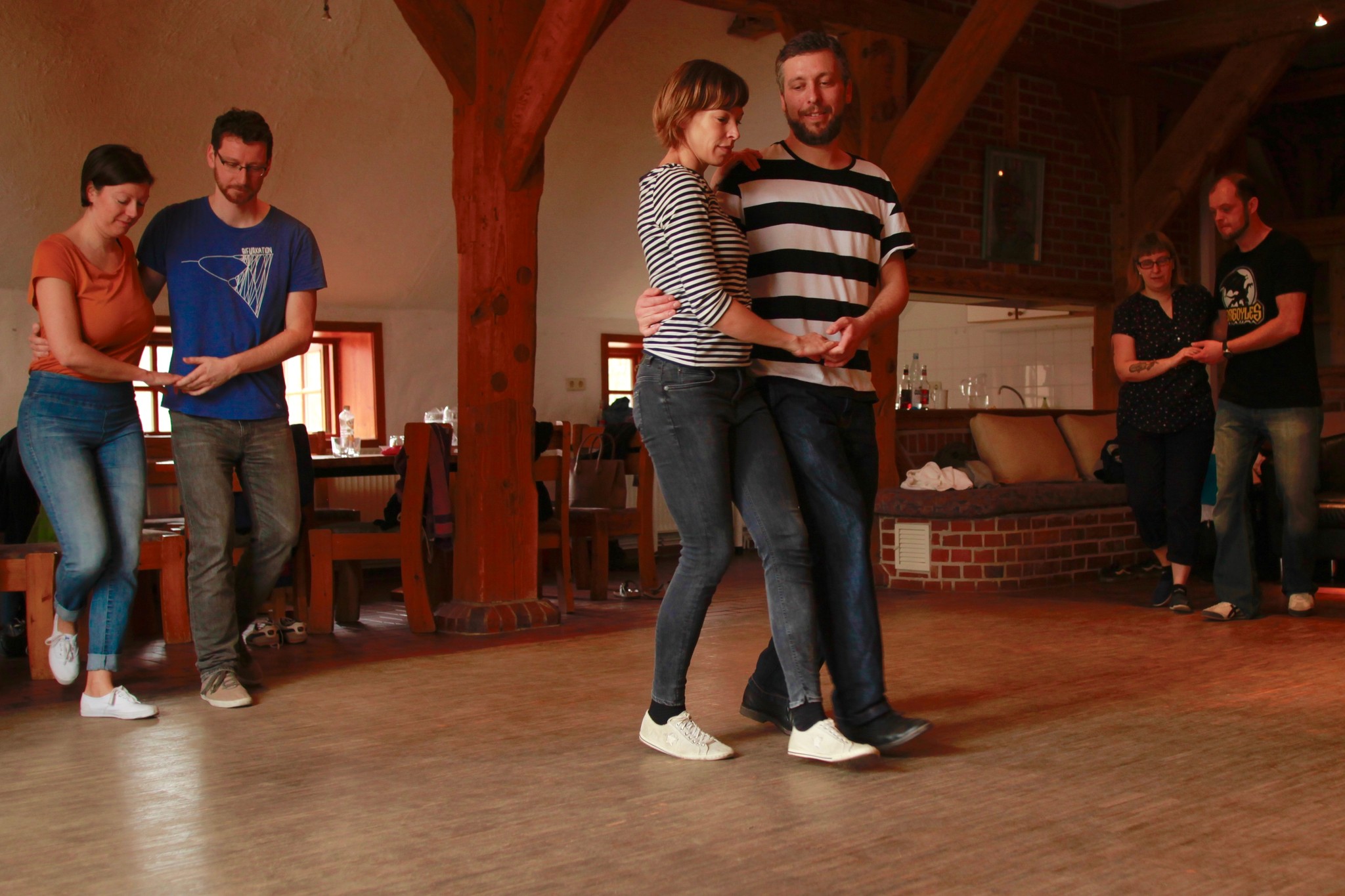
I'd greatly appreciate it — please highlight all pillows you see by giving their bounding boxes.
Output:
[1057,413,1120,481]
[970,414,1082,484]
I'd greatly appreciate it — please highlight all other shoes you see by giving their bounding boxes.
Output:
[1152,566,1174,607]
[1171,584,1193,612]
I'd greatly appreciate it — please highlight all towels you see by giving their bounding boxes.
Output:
[900,460,975,492]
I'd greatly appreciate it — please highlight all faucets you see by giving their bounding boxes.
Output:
[997,385,1028,408]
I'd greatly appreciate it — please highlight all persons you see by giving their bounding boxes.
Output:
[633,60,880,761]
[1192,173,1323,619]
[17,144,186,719]
[1112,231,1228,609]
[635,35,935,749]
[29,109,328,707]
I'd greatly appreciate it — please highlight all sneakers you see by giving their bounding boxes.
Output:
[45,612,79,685]
[787,717,879,763]
[231,634,264,683]
[1201,600,1246,620]
[639,709,735,760]
[81,685,159,719]
[1286,591,1314,611]
[200,669,252,708]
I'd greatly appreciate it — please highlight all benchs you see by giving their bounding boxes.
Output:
[874,409,1139,597]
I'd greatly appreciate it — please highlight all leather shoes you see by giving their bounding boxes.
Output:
[841,711,933,752]
[740,684,796,733]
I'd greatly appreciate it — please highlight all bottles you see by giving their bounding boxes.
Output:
[390,435,405,448]
[899,364,913,410]
[912,352,922,410]
[920,365,929,410]
[1041,397,1049,409]
[338,405,354,458]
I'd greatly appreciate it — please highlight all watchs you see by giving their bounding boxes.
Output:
[1222,342,1233,359]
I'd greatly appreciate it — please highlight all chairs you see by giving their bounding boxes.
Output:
[0,421,656,680]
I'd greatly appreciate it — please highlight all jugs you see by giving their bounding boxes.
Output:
[959,373,989,409]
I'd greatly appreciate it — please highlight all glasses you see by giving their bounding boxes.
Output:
[1139,256,1173,269]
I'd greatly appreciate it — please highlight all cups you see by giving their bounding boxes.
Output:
[332,436,340,456]
[354,438,361,457]
[930,389,948,410]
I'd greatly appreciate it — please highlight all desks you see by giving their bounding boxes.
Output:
[152,453,450,473]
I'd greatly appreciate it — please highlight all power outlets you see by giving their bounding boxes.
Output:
[566,378,588,391]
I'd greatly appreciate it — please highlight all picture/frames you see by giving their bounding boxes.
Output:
[981,145,1047,265]
